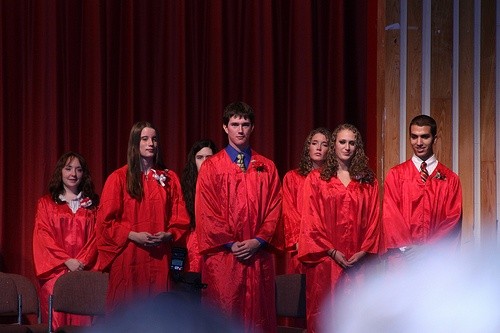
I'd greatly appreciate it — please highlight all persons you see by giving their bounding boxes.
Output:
[195,101,282,333]
[96,121,190,314]
[282,127,336,330]
[293,124,382,333]
[180,138,221,293]
[33,150,104,333]
[381,115,463,259]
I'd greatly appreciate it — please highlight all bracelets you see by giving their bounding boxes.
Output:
[331,249,337,259]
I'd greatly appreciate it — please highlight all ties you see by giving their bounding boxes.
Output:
[236,154,245,172]
[420,162,430,184]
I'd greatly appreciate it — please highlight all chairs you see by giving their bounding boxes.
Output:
[0,272,108,333]
[275,274,306,333]
